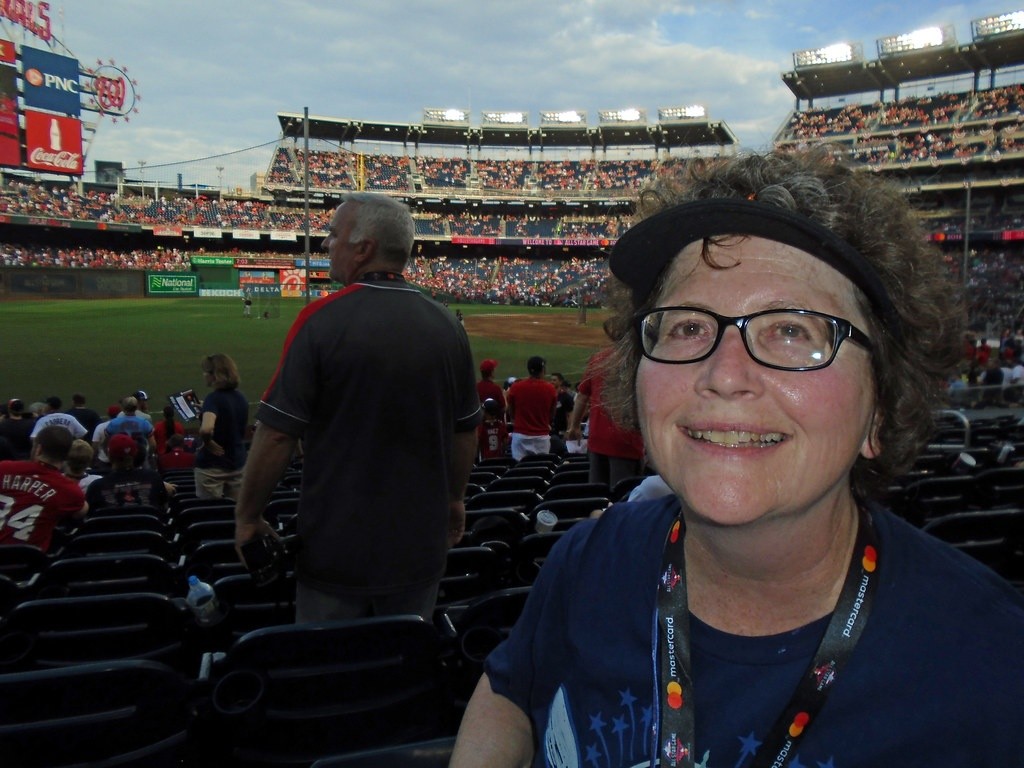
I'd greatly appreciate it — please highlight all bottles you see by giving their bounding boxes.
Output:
[185,575,217,623]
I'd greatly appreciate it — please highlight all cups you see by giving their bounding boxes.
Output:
[952,452,976,474]
[536,509,558,534]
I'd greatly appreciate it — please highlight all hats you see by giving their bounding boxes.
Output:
[480,360,498,372]
[107,434,138,458]
[608,197,903,348]
[133,391,148,400]
[527,357,545,373]
[1003,348,1013,359]
[107,405,122,414]
[481,397,499,414]
[976,351,988,363]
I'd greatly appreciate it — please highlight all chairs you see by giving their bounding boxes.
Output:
[0,413,1024,768]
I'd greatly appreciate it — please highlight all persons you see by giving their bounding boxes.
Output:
[236,192,484,627]
[445,146,1024,768]
[1,82,1022,598]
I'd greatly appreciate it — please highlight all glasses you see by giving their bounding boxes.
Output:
[630,305,878,371]
